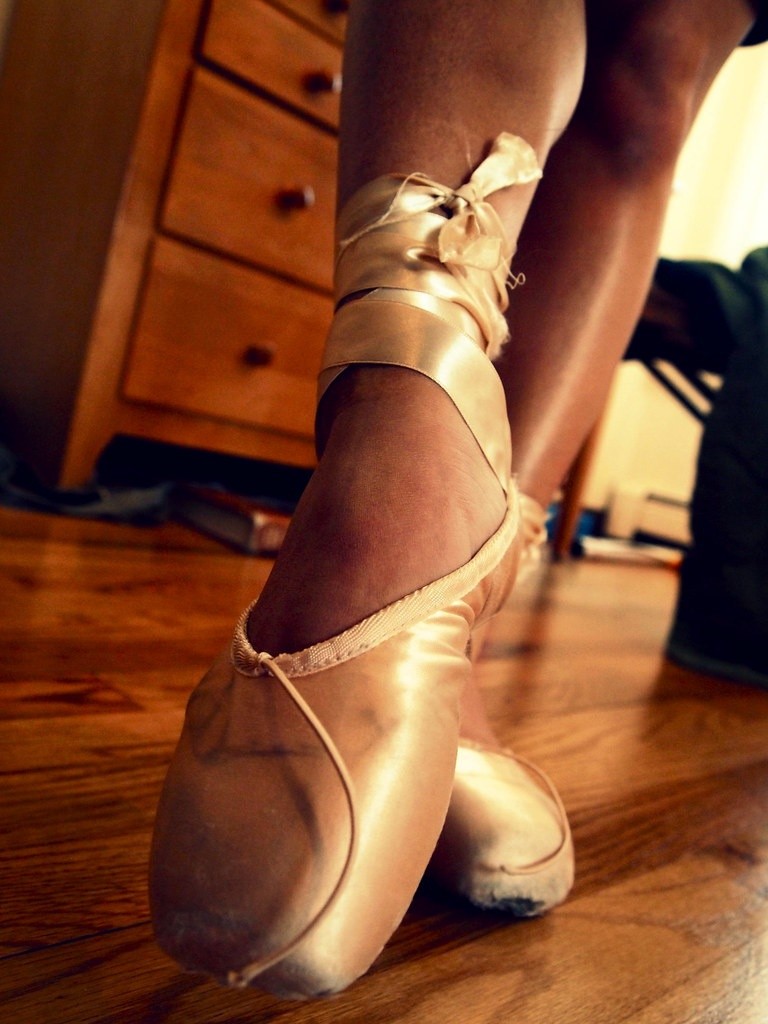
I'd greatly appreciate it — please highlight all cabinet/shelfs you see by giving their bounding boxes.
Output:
[0,0,343,497]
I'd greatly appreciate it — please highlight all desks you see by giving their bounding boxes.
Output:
[556,286,723,559]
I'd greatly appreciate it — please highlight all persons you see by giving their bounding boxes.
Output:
[116,0,768,1002]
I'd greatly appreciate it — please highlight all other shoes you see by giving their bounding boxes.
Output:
[666,602,768,686]
[429,739,575,918]
[149,129,545,1002]
